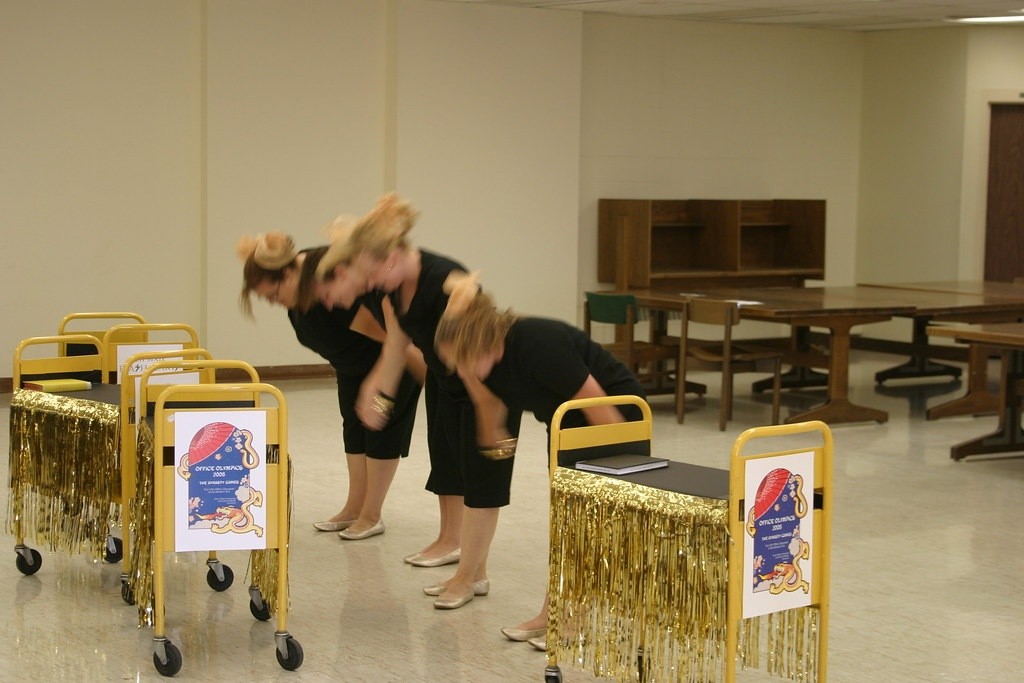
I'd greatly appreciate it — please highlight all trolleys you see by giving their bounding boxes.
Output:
[543,394,833,683]
[8,312,306,675]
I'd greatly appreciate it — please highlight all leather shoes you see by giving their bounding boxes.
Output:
[424,577,489,596]
[411,547,460,567]
[433,590,474,609]
[501,626,547,641]
[339,518,384,540]
[313,519,356,531]
[404,552,421,564]
[527,637,547,651]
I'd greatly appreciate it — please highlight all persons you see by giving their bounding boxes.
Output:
[238,231,422,540]
[347,201,523,608]
[432,297,651,654]
[296,239,464,567]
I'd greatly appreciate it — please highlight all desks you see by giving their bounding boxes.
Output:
[926,324,1024,462]
[856,279,1024,385]
[752,286,1014,421]
[598,288,916,426]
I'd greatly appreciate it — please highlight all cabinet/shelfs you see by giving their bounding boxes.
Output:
[597,198,828,373]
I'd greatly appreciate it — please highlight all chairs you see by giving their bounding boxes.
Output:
[675,296,783,432]
[582,290,678,385]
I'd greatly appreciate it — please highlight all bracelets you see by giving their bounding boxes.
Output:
[372,389,395,416]
[480,439,516,459]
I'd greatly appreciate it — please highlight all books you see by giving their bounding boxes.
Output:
[21,378,93,392]
[574,453,669,476]
